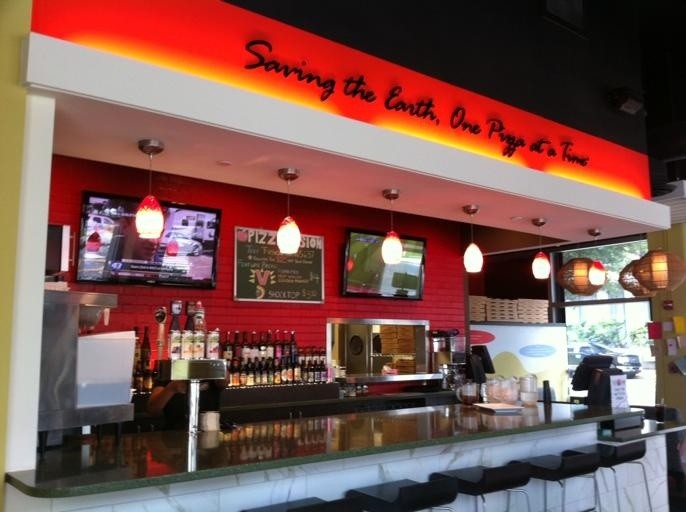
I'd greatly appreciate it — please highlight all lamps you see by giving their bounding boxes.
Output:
[381,188,403,266]
[463,204,484,273]
[557,230,685,298]
[135,138,166,240]
[276,167,303,255]
[530,217,551,280]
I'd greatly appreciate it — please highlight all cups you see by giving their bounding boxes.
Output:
[456,374,538,407]
[430,407,539,439]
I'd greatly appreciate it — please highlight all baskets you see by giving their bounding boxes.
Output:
[518,299,549,323]
[470,296,486,321]
[486,298,518,322]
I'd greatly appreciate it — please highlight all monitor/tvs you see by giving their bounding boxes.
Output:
[471,344,496,374]
[75,189,222,291]
[342,227,427,301]
[571,353,613,390]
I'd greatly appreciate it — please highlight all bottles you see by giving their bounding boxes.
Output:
[131,299,370,399]
[80,417,349,472]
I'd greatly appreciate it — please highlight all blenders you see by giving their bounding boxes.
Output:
[431,329,460,389]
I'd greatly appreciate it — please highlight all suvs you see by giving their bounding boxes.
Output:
[557,341,643,379]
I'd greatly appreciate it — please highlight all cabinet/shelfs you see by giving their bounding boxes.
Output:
[36,289,144,430]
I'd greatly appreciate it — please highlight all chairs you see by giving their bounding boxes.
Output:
[630,405,680,474]
